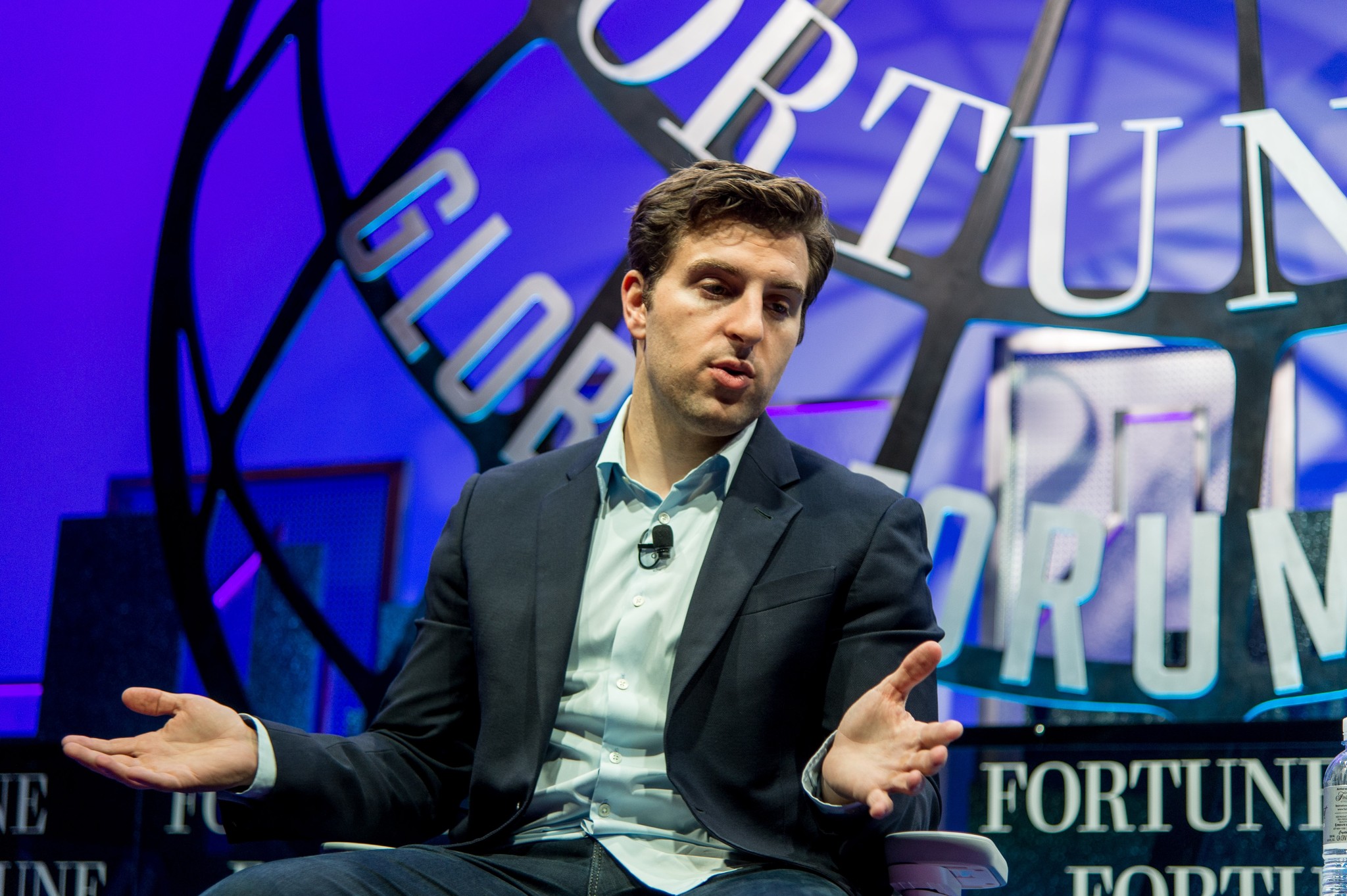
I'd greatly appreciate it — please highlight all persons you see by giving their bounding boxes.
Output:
[61,156,964,895]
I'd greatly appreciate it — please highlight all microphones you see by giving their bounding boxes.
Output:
[652,524,675,560]
